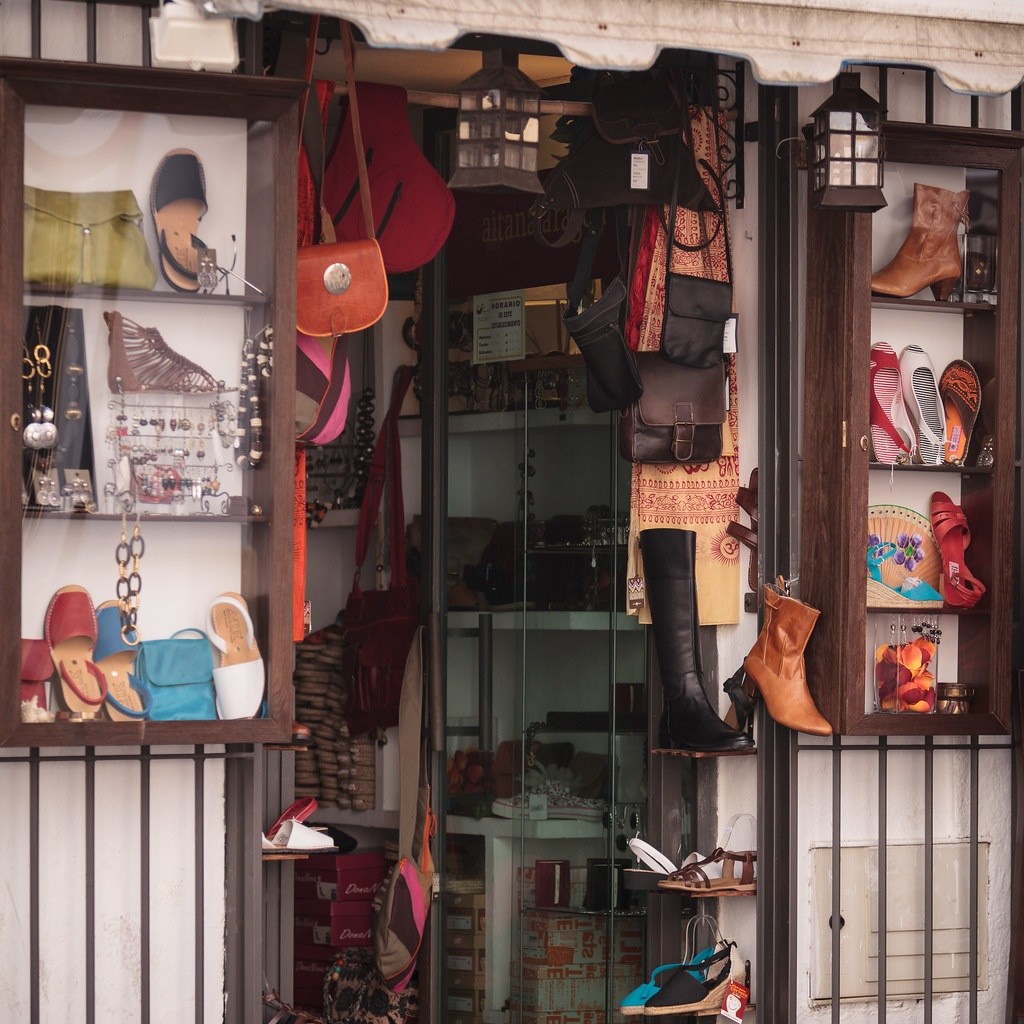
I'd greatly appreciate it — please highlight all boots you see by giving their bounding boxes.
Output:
[869,183,970,302]
[637,529,755,751]
[734,584,833,737]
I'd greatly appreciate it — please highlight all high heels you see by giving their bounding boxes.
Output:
[644,937,746,1015]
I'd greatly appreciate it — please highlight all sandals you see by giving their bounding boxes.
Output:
[870,341,917,467]
[622,837,740,890]
[261,816,340,853]
[150,147,214,292]
[107,311,219,394]
[900,344,948,466]
[205,591,265,719]
[657,847,758,892]
[930,491,986,609]
[45,583,107,719]
[94,600,153,722]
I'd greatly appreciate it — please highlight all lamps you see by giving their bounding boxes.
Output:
[442,46,547,197]
[801,62,890,211]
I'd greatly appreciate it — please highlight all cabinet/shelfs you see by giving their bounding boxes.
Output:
[1,65,1024,1023]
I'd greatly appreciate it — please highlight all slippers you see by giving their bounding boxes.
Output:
[938,360,981,467]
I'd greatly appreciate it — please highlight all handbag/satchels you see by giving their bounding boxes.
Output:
[134,630,221,720]
[273,14,390,446]
[554,150,737,466]
[343,593,419,732]
[21,185,157,291]
[377,855,431,994]
[21,636,51,716]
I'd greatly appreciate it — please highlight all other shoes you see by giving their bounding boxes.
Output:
[620,945,713,1015]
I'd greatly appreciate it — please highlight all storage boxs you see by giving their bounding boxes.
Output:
[292,944,343,990]
[292,899,377,948]
[444,934,488,974]
[441,893,485,935]
[297,848,380,900]
[441,974,488,1016]
